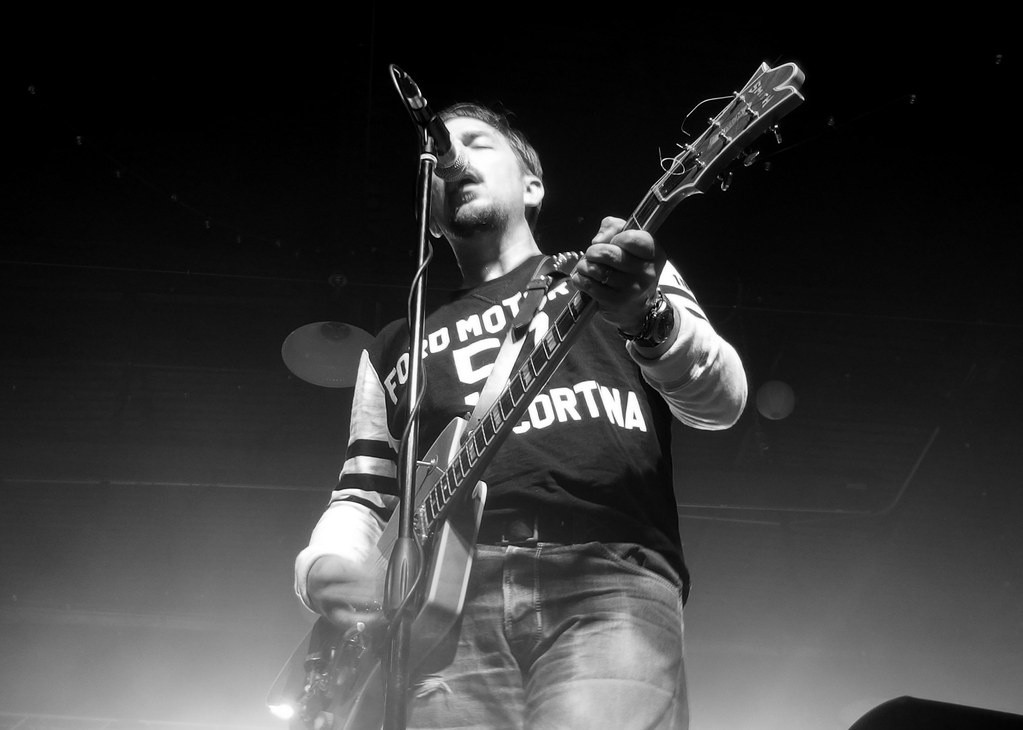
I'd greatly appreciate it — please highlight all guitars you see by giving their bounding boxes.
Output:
[266,58,806,730]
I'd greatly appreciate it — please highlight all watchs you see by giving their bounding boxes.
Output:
[633,291,675,350]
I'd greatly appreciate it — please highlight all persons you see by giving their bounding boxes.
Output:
[291,103,750,730]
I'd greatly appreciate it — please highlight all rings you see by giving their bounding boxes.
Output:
[600,266,615,285]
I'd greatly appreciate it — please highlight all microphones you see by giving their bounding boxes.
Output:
[399,72,470,184]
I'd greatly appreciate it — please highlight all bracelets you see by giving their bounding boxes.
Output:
[615,291,664,341]
